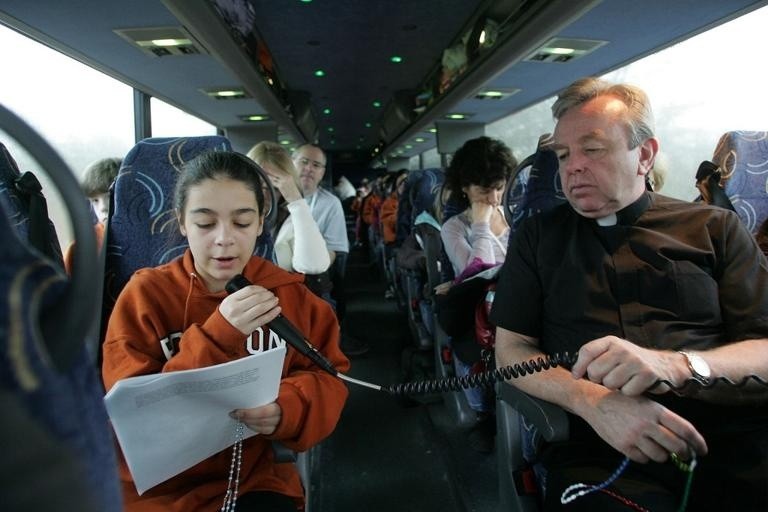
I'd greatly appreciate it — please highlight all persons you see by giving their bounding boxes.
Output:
[441,136,519,454]
[63,156,123,283]
[345,167,451,337]
[242,141,330,281]
[289,143,349,268]
[94,150,350,512]
[497,75,767,511]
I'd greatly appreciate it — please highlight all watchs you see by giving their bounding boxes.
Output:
[679,351,712,385]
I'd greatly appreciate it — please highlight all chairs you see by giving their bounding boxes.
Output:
[1,106,122,512]
[713,132,767,236]
[108,136,279,310]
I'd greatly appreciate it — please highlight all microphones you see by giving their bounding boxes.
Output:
[225,273,335,381]
